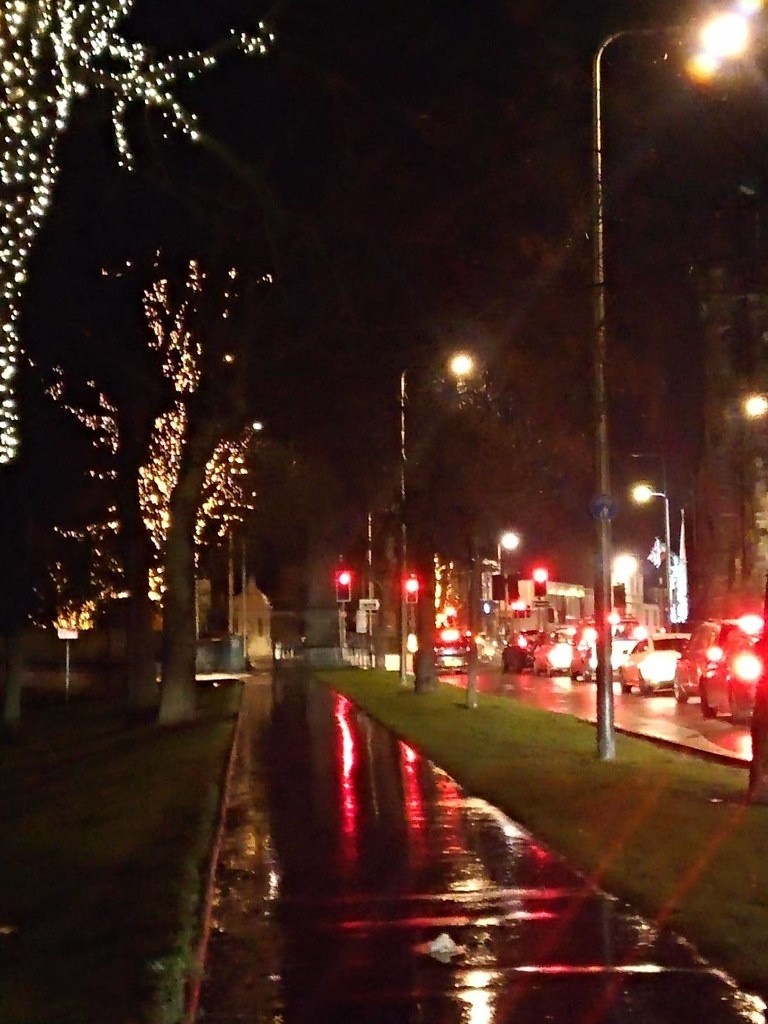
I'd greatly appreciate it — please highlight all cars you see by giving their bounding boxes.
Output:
[619,617,764,723]
[502,624,637,683]
[413,628,503,675]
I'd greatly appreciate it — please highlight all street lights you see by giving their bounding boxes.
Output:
[498,533,521,649]
[631,450,668,498]
[633,486,672,633]
[590,13,752,763]
[400,340,476,687]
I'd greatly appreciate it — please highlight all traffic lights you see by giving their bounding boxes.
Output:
[335,570,352,603]
[534,569,548,596]
[406,576,419,605]
